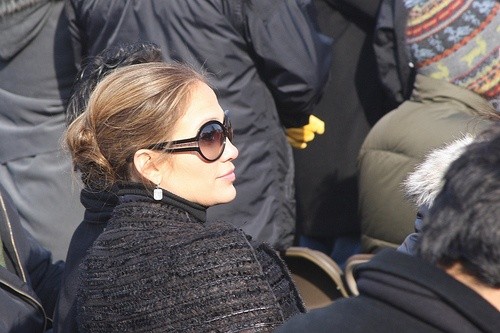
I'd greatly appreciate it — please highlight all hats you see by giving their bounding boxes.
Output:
[402,0,500,100]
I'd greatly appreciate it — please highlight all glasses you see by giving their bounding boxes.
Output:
[140,109,233,163]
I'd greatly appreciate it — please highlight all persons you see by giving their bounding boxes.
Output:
[66,62,305,333]
[0,0,500,333]
